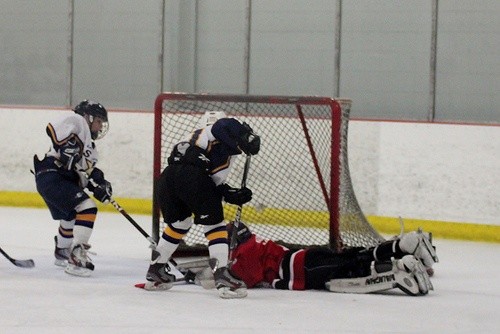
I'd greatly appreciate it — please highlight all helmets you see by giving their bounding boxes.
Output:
[73,99,110,140]
[228,220,252,244]
[203,110,226,126]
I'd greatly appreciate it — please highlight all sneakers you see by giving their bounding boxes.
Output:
[209,257,248,299]
[415,230,439,276]
[54,235,72,267]
[65,243,95,276]
[145,250,176,290]
[400,254,434,294]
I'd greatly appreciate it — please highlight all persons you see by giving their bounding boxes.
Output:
[146,111,261,296]
[32,99,110,276]
[207,218,439,297]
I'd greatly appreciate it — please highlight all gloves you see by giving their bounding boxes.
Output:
[87,168,112,203]
[240,122,261,156]
[60,137,83,164]
[223,186,252,205]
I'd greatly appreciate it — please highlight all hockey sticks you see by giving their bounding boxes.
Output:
[0,248,34,267]
[228,134,254,270]
[76,162,195,285]
